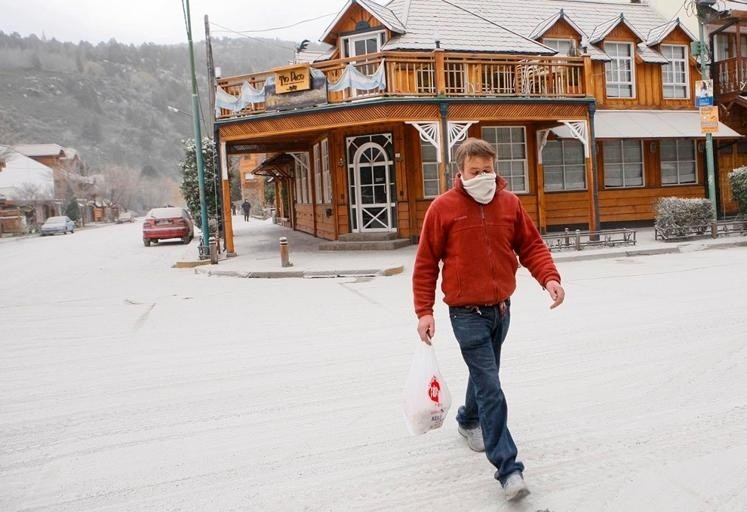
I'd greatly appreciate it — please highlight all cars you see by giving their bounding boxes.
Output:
[142,207,194,247]
[118,212,135,224]
[40,216,75,235]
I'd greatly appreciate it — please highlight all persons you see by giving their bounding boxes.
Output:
[700,82,707,97]
[231,202,236,216]
[412,138,565,502]
[242,200,251,222]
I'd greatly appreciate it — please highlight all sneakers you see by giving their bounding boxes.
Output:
[503,475,529,501]
[457,426,485,452]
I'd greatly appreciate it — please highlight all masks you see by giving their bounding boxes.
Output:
[460,173,497,204]
